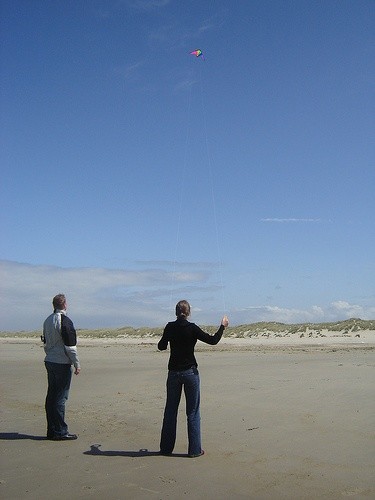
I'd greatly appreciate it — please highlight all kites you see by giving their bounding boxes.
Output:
[189,49,206,62]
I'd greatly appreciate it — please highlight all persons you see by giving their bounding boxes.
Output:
[40,294,81,441]
[157,300,229,458]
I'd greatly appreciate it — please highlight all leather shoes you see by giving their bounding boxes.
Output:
[47,434,77,440]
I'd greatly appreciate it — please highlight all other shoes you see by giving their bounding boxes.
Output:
[188,450,204,458]
[160,448,172,456]
